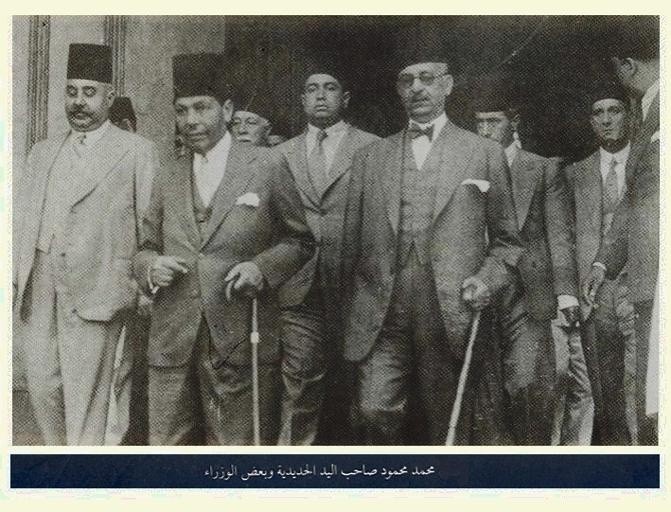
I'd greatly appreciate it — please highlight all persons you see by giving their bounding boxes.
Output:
[14,29,660,445]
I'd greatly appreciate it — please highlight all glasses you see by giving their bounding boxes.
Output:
[399,68,449,91]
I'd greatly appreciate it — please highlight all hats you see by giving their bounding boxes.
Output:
[234,78,276,123]
[469,75,512,115]
[393,23,455,77]
[65,43,114,84]
[170,51,227,103]
[590,77,629,108]
[108,95,136,128]
[299,51,352,93]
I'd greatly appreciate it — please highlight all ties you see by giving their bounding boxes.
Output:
[600,157,619,228]
[309,127,330,197]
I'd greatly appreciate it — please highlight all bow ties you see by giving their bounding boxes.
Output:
[406,122,436,140]
